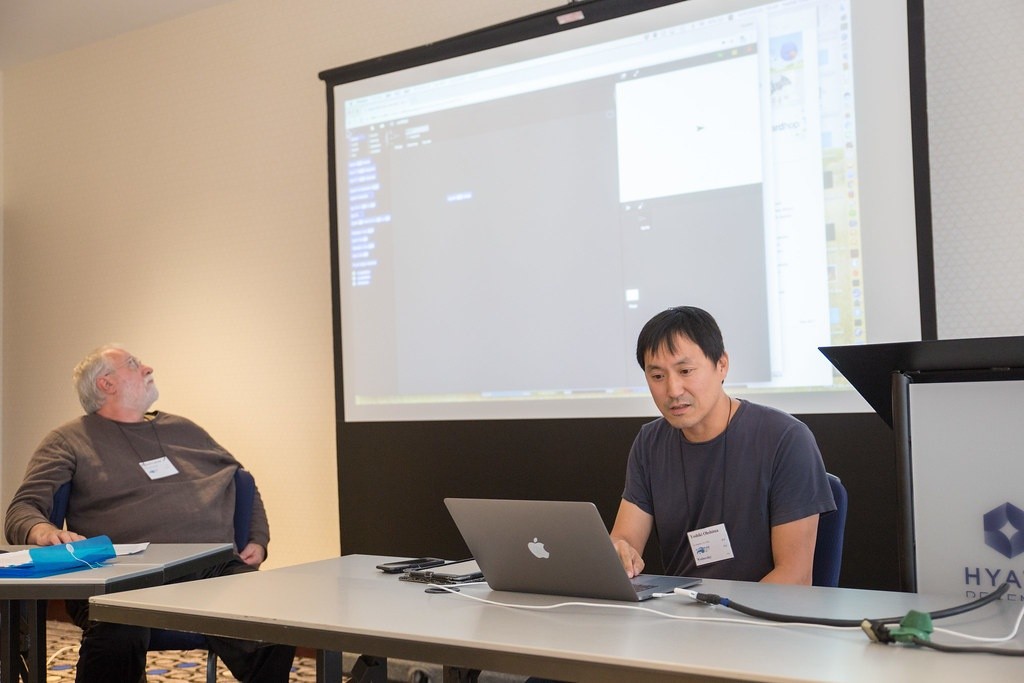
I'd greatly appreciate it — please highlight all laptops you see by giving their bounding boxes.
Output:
[444,497,703,602]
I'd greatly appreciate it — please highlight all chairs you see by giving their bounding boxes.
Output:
[148,466,254,683]
[813,468,846,591]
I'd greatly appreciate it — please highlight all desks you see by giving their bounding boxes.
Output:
[90,552,1024,682]
[1,537,232,683]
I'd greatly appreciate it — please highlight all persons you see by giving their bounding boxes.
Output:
[3,348,299,683]
[608,305,839,587]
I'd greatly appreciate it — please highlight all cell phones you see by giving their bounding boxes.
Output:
[376,558,444,570]
